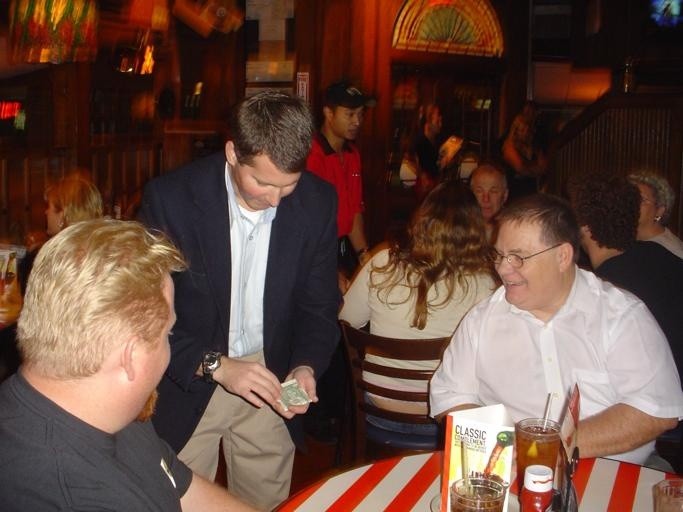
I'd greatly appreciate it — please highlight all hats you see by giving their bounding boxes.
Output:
[322,81,378,109]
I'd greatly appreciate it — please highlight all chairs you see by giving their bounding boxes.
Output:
[339,319,450,464]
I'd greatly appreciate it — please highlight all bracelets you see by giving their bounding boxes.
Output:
[355,247,370,258]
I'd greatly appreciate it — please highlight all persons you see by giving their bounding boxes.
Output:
[424,194,683,471]
[129,88,347,511]
[409,104,445,200]
[499,108,550,193]
[0,215,256,511]
[566,174,683,388]
[13,171,103,289]
[627,168,683,265]
[469,164,508,222]
[293,78,371,442]
[327,173,505,455]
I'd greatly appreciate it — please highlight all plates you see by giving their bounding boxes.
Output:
[429,491,521,512]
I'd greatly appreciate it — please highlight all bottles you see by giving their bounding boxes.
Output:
[515,465,552,512]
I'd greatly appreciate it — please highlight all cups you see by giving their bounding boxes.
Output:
[514,420,561,489]
[451,475,505,512]
[655,479,683,512]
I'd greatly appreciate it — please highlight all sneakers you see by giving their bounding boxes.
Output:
[313,427,341,447]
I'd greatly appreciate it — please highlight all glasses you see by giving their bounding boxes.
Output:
[484,242,565,269]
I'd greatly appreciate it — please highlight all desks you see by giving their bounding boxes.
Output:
[270,449,683,512]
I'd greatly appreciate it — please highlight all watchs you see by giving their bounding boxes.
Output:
[200,349,224,383]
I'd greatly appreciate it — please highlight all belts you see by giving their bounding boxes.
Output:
[338,236,351,258]
